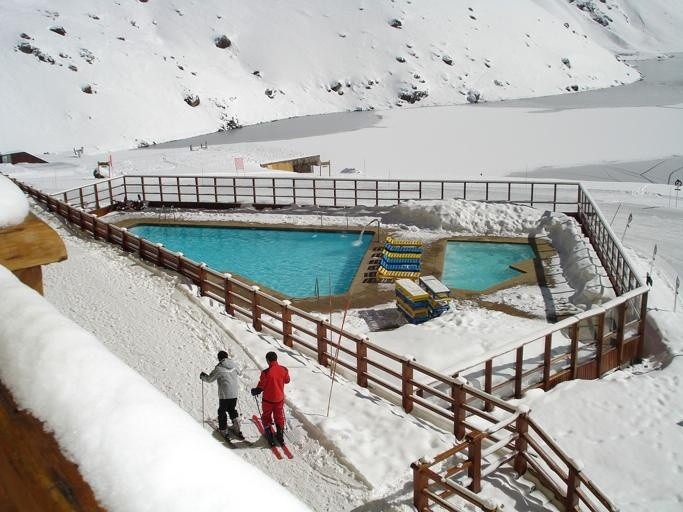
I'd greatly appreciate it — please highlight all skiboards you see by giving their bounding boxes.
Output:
[204,419,253,448]
[252,414,293,459]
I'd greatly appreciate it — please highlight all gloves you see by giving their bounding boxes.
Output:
[251,387,261,396]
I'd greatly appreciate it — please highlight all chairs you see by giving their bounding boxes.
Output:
[377,235,424,284]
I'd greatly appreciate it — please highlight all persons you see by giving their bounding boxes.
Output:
[251,352,291,447]
[200,351,241,438]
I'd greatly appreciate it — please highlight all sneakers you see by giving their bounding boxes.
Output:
[216,420,241,437]
[265,432,285,447]
